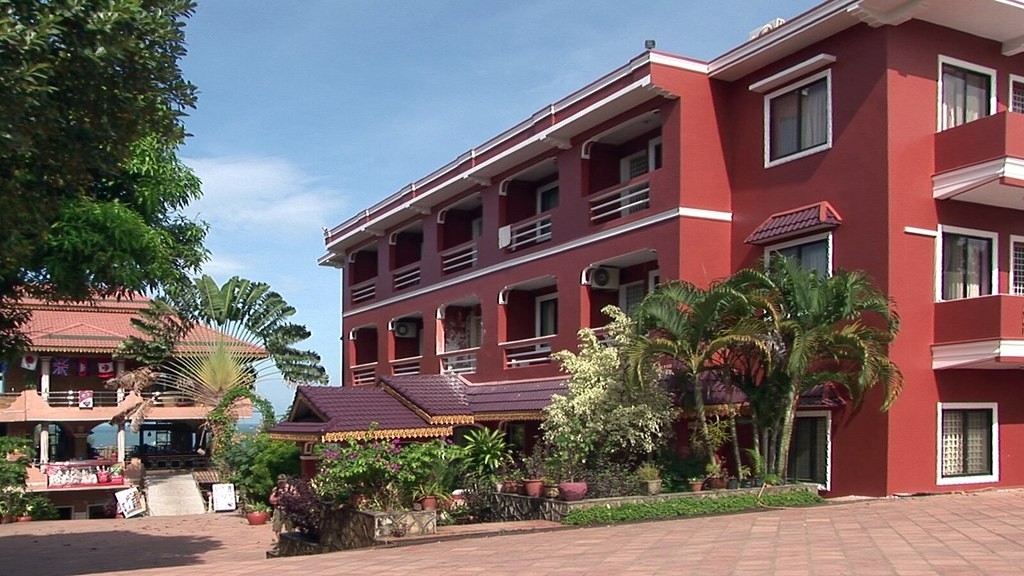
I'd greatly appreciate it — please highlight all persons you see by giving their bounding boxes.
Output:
[269,473,298,545]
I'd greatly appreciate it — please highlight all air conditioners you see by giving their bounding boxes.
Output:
[392,320,418,339]
[590,266,621,290]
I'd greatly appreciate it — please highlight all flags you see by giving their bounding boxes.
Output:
[97,360,114,378]
[51,357,69,375]
[20,353,38,370]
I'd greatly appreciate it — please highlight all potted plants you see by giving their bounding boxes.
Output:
[556,474,588,502]
[96,462,123,484]
[244,501,270,526]
[523,473,545,496]
[545,480,559,498]
[500,471,519,494]
[687,476,704,491]
[706,464,726,488]
[0,483,52,522]
[727,474,739,490]
[413,481,446,512]
[739,464,752,490]
[636,460,664,497]
